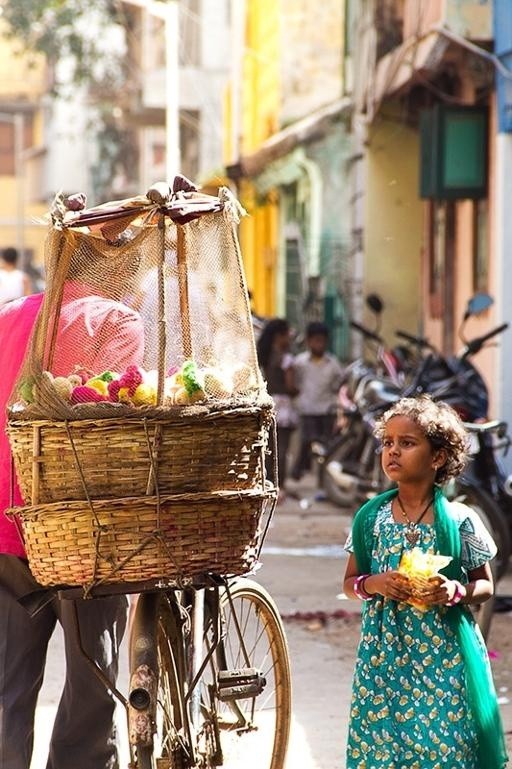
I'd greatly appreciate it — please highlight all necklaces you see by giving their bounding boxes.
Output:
[397,494,434,550]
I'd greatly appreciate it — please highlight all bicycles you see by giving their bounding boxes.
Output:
[58,579,291,769]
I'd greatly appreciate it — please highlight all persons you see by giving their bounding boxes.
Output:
[344,393,510,769]
[0,248,33,306]
[287,322,353,499]
[0,227,145,769]
[255,319,301,507]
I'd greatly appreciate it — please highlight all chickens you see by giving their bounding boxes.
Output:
[19,357,252,407]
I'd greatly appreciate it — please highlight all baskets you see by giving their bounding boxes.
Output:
[4,396,280,588]
[4,480,273,585]
[4,396,274,506]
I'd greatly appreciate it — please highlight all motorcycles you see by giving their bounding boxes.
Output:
[319,292,512,579]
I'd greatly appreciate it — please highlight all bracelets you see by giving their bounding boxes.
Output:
[446,580,463,607]
[353,574,374,601]
